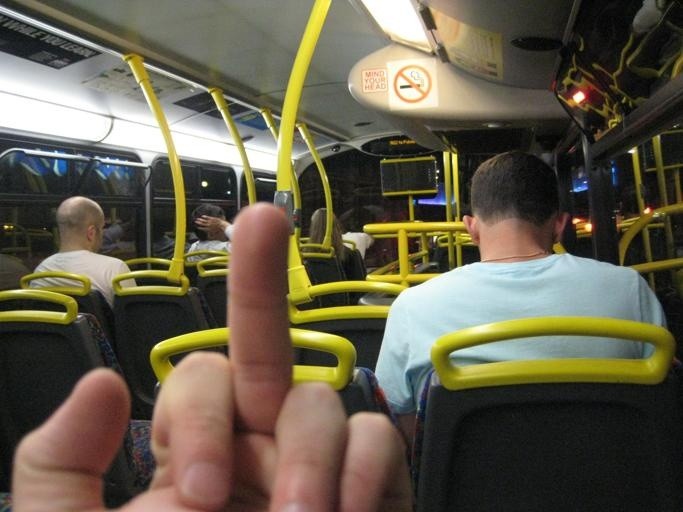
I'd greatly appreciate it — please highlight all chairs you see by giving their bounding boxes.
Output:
[0,217,683,511]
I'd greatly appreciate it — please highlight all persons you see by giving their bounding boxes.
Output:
[28,195,138,309]
[5,200,415,512]
[301,207,367,281]
[368,147,670,455]
[187,201,232,261]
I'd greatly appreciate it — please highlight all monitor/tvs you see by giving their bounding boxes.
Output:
[381,155,439,197]
